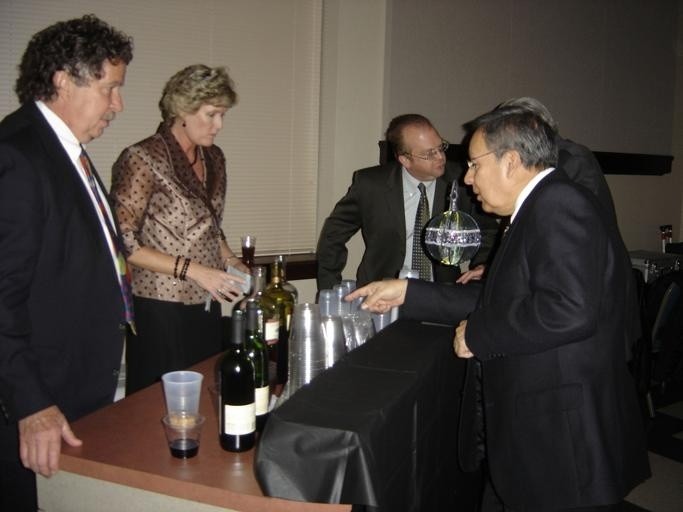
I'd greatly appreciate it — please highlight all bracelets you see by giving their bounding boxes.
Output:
[220,255,238,269]
[173,255,193,279]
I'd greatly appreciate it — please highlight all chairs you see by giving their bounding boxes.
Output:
[646,282,677,418]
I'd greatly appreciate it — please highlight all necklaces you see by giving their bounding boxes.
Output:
[188,146,199,165]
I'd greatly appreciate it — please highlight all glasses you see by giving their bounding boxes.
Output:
[403,138,449,162]
[468,150,499,168]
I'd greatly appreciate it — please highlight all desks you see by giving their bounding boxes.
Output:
[37,304,352,512]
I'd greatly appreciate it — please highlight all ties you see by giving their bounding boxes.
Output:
[80,146,140,337]
[412,184,432,284]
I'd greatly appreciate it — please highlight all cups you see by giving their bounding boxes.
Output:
[161,370,205,433]
[161,410,204,460]
[286,269,423,401]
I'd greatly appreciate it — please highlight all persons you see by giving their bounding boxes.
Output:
[114,63,254,397]
[456,99,644,359]
[0,15,136,512]
[345,106,652,512]
[315,111,487,302]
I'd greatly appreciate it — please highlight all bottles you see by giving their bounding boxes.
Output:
[235,269,279,349]
[220,311,256,452]
[242,299,269,430]
[277,256,299,309]
[264,260,293,336]
